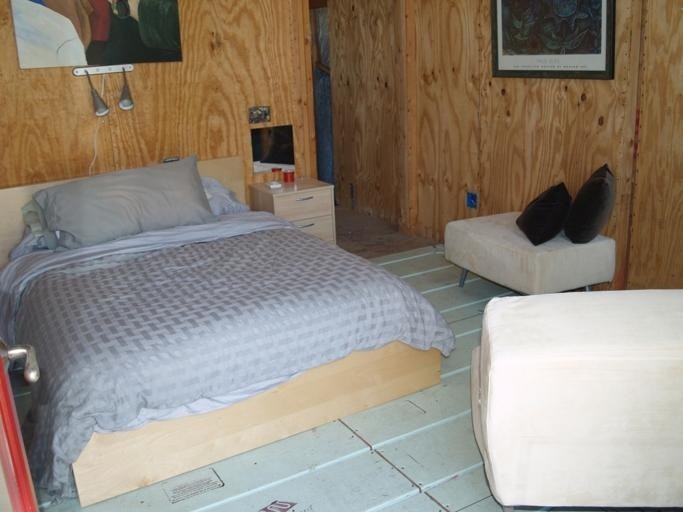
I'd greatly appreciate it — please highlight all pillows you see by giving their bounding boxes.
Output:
[9,156,246,262]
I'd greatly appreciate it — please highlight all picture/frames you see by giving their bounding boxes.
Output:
[491,2,615,81]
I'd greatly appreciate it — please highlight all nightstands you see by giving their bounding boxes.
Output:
[249,175,337,246]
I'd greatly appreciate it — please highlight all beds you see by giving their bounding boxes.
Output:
[0,156,458,509]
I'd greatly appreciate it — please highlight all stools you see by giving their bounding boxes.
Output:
[442,210,617,296]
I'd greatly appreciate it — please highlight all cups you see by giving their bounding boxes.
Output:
[271,168,282,185]
[281,170,294,184]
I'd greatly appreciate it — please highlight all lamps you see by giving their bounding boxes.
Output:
[84,67,135,117]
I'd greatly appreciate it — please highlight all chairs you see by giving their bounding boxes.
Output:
[468,287,683,510]
